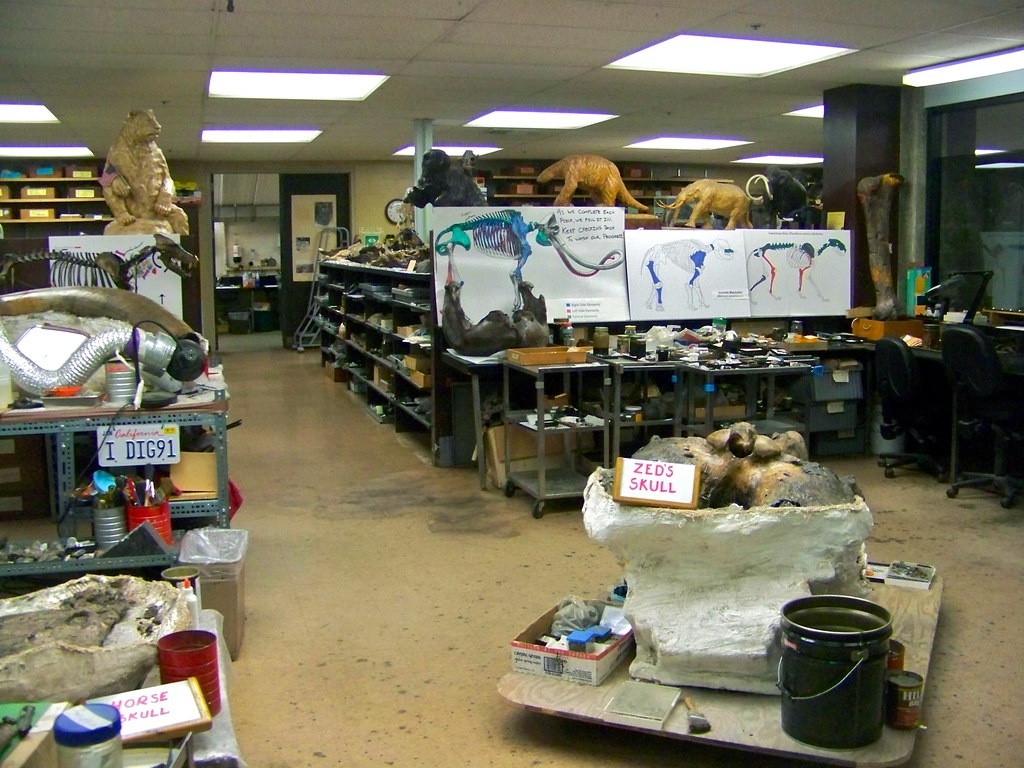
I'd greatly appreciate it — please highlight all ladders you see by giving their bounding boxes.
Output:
[292,227,350,352]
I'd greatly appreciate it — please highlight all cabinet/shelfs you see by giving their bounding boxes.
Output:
[0,369,230,577]
[314,260,452,467]
[0,158,114,224]
[502,346,810,519]
[489,157,735,225]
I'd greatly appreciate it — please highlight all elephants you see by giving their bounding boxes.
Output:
[656,165,823,230]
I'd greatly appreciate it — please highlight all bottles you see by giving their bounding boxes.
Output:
[177,578,200,631]
[560,322,575,348]
[53,703,124,768]
[592,326,668,360]
[242,271,260,286]
[791,320,802,335]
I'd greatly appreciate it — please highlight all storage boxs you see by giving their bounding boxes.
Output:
[371,319,431,395]
[669,400,744,423]
[625,211,660,230]
[170,433,228,503]
[0,165,104,218]
[325,360,345,382]
[472,424,701,510]
[511,597,635,687]
[786,359,865,458]
[508,165,685,196]
[849,316,924,340]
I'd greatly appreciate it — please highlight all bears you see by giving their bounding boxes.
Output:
[101,108,190,237]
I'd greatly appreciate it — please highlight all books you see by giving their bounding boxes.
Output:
[865,560,936,590]
[603,681,682,730]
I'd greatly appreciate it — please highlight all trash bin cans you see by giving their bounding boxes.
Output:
[227,307,252,334]
[179,528,249,662]
[254,302,274,332]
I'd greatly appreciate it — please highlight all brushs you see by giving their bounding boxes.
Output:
[684,695,710,734]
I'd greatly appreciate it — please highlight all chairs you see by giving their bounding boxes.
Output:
[874,336,948,483]
[941,324,1024,508]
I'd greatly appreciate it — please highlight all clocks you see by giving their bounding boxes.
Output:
[385,199,407,225]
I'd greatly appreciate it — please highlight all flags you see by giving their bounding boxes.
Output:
[98,166,118,187]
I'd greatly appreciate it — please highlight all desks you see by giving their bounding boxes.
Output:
[216,284,279,332]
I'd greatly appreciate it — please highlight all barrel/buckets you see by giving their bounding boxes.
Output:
[92,501,172,553]
[104,360,139,406]
[774,595,893,752]
[887,637,924,729]
[161,566,204,617]
[156,629,224,720]
